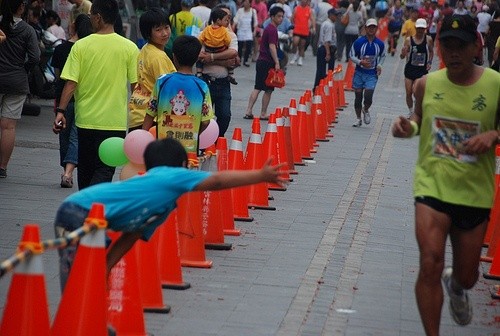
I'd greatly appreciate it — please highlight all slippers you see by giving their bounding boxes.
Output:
[243,114,254,119]
[259,117,269,120]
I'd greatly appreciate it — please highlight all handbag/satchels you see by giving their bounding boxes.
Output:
[266,69,285,89]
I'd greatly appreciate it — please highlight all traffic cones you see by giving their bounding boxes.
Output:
[262,98,306,191]
[0,224,51,336]
[135,117,277,315]
[480,144,500,299]
[51,203,148,336]
[297,59,355,160]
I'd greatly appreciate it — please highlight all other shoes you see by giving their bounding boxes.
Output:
[408,111,414,118]
[391,49,396,56]
[227,75,238,85]
[0,168,7,178]
[196,73,203,79]
[298,57,303,66]
[60,175,73,188]
[388,48,391,53]
[290,57,298,64]
[244,62,250,66]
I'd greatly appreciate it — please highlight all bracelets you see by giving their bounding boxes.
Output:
[409,121,418,137]
[210,53,214,62]
[56,107,66,114]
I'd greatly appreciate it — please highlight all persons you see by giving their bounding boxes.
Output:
[253,0,500,74]
[128,11,178,134]
[142,35,219,174]
[0,0,40,178]
[399,18,434,119]
[0,0,124,98]
[233,0,258,67]
[289,0,316,66]
[51,0,141,191]
[54,13,91,188]
[392,15,500,336]
[54,137,294,336]
[195,4,238,139]
[349,18,386,128]
[21,0,239,101]
[196,8,239,85]
[243,6,288,121]
[313,8,341,95]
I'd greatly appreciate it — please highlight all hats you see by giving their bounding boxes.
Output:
[483,4,489,12]
[415,18,427,28]
[327,8,340,15]
[439,13,479,40]
[366,18,378,27]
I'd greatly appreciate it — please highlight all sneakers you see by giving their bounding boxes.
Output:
[443,267,473,326]
[352,119,362,127]
[362,107,371,124]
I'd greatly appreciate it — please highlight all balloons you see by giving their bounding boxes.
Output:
[120,163,138,180]
[199,119,219,150]
[98,137,130,167]
[122,129,156,164]
[149,125,156,138]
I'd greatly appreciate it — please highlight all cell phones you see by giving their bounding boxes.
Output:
[56,121,63,133]
